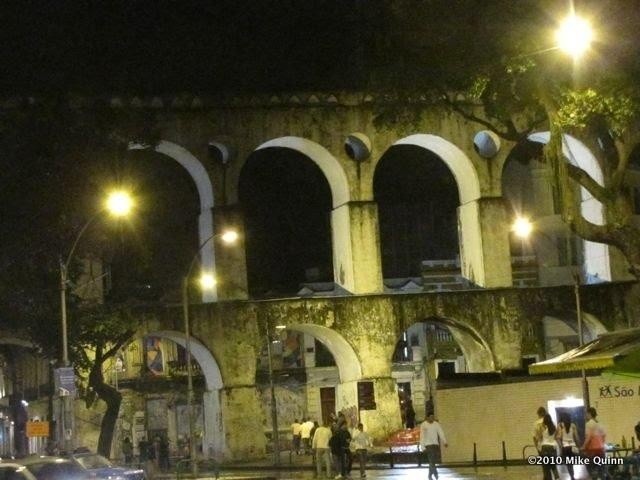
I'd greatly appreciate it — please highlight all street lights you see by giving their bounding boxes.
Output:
[180,228,238,479]
[512,217,591,408]
[58,190,132,457]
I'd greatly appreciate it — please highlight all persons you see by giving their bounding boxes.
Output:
[291,410,374,479]
[538,415,561,480]
[118,428,173,473]
[111,353,126,372]
[557,412,582,480]
[419,411,448,480]
[580,407,609,480]
[533,405,551,478]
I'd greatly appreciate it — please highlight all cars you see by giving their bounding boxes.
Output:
[0,445,149,480]
